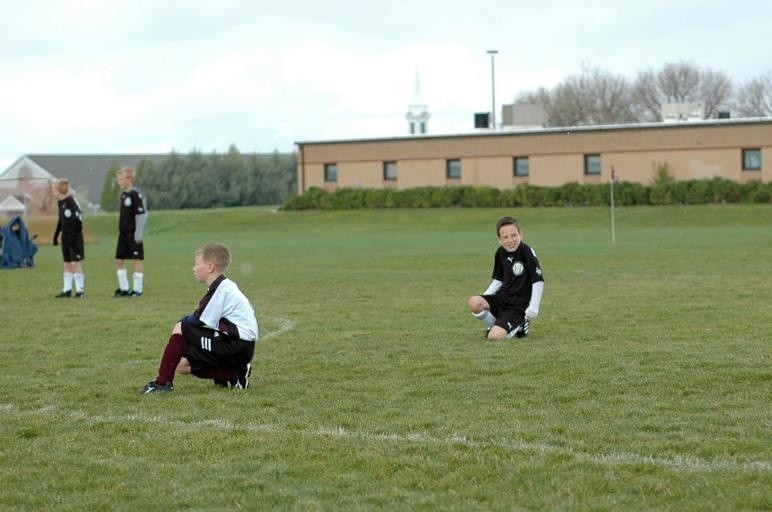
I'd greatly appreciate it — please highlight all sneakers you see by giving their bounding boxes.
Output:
[113,289,143,297]
[226,363,251,392]
[136,381,175,395]
[484,328,491,338]
[56,290,84,298]
[516,318,528,339]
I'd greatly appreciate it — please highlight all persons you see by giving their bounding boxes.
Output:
[467,216,545,342]
[1,216,38,270]
[111,166,149,299]
[133,241,259,395]
[51,179,87,299]
